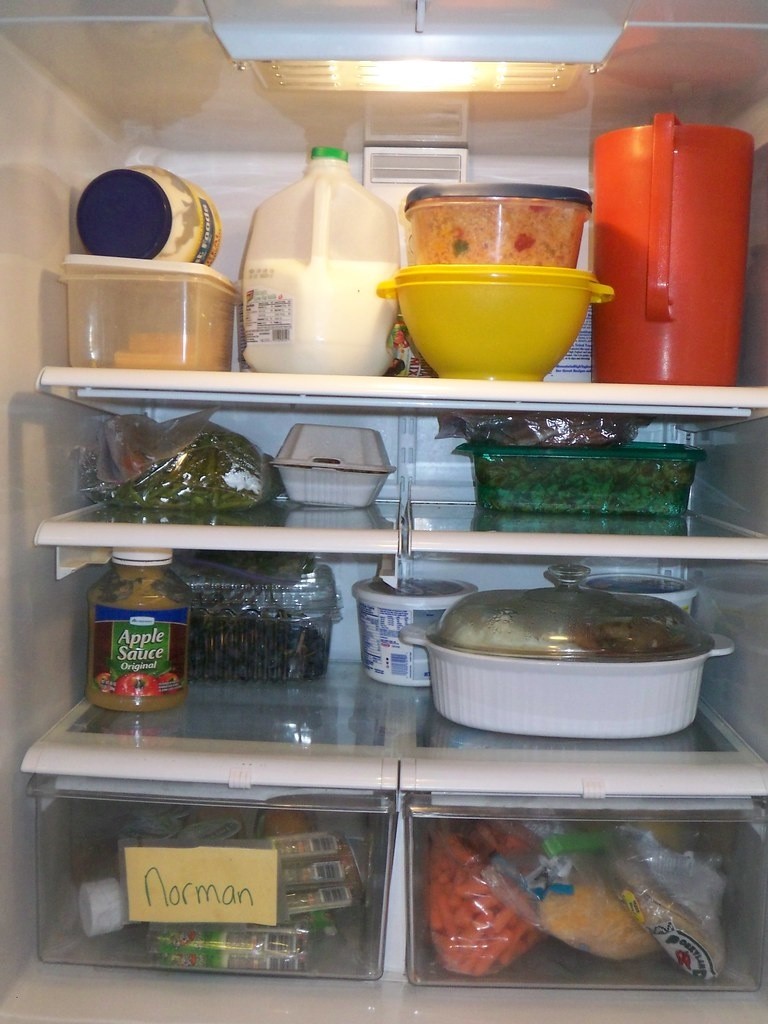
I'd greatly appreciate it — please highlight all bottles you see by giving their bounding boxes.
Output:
[89,542,189,712]
[77,164,222,267]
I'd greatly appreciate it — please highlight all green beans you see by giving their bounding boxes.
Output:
[74,422,284,510]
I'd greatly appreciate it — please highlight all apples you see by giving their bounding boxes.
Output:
[94,672,181,697]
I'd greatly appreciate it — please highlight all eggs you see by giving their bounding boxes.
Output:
[267,809,310,841]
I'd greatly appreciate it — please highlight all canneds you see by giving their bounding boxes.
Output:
[77,164,220,268]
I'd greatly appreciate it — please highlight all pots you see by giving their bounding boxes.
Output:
[404,561,738,739]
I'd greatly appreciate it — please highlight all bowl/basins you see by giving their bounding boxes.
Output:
[577,575,700,622]
[351,578,479,685]
[399,183,594,269]
[454,444,707,515]
[57,254,244,371]
[269,424,395,508]
[374,265,615,381]
[177,556,343,685]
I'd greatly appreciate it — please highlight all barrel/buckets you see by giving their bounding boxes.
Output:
[235,147,401,374]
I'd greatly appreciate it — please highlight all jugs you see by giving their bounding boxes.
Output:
[592,113,755,386]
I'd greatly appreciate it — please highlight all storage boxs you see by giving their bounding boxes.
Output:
[59,253,243,372]
[455,444,707,517]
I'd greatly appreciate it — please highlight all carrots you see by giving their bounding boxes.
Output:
[422,822,561,975]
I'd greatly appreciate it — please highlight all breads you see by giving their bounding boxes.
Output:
[612,858,726,977]
